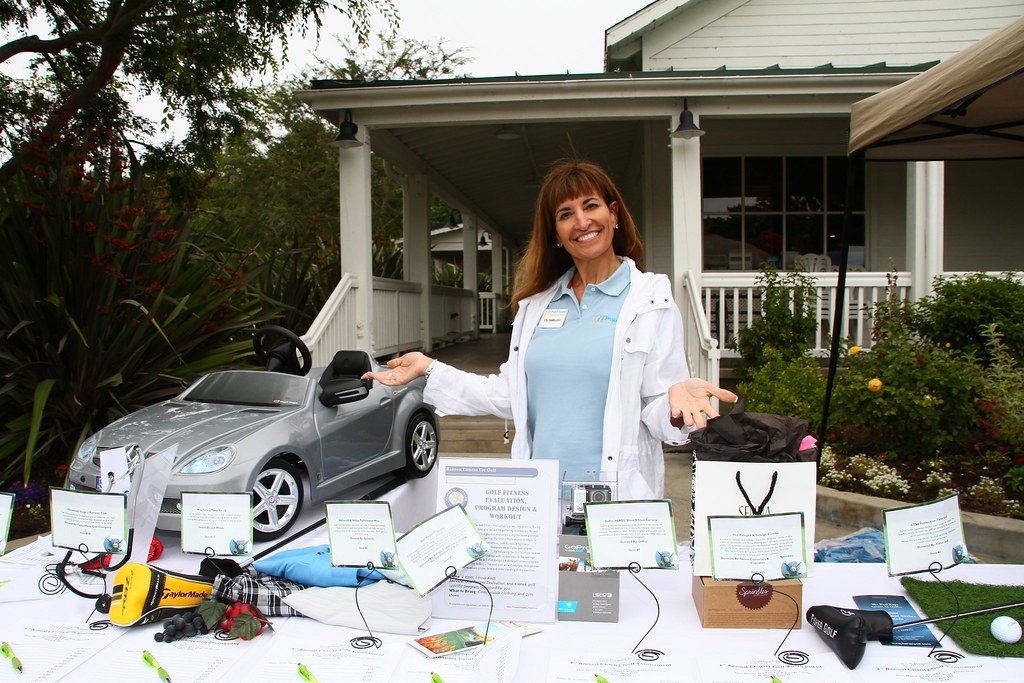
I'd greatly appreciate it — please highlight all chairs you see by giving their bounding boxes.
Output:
[318,350,373,392]
[793,253,870,322]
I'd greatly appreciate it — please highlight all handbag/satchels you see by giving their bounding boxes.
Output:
[689,452,817,577]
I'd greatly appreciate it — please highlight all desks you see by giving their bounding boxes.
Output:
[57,531,1024,683]
[0,462,437,683]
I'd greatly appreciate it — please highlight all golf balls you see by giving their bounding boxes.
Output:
[990,615,1023,644]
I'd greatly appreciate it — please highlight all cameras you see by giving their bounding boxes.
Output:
[566,485,612,517]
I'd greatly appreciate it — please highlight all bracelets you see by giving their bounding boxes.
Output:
[424,358,438,382]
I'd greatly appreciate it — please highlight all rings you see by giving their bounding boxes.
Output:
[389,373,394,377]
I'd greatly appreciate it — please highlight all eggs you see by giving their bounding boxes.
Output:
[990,616,1022,644]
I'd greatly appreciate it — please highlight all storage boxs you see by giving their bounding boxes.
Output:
[558,469,620,623]
[691,572,803,628]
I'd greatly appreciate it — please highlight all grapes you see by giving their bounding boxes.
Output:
[154,611,211,643]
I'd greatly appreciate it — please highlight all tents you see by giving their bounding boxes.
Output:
[815,14,1024,477]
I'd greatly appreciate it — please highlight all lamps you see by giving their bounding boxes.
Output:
[443,209,462,229]
[478,231,491,247]
[329,109,363,149]
[668,97,706,140]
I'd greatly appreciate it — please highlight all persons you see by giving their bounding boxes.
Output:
[362,161,738,502]
